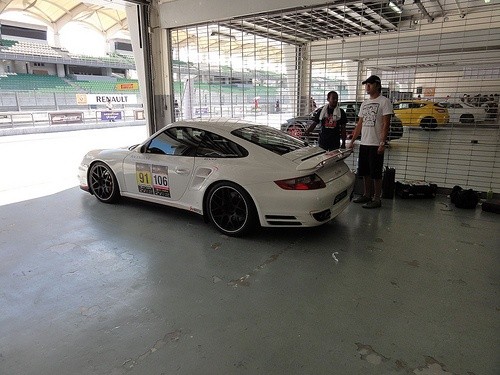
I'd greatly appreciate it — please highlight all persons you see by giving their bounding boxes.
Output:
[444,95,449,102]
[310,97,316,112]
[416,95,421,100]
[252,100,261,110]
[275,101,279,113]
[461,93,500,118]
[303,91,346,152]
[348,75,394,210]
[173,99,179,118]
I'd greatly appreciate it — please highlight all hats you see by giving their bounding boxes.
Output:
[361,74,382,85]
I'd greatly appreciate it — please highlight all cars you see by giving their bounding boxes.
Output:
[391,99,488,131]
[77,121,356,236]
[280,101,403,139]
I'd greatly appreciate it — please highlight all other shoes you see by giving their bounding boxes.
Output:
[361,199,382,209]
[353,195,373,203]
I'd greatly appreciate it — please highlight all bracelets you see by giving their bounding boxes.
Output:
[379,141,385,145]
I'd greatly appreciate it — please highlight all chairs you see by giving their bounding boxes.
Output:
[0,38,125,94]
